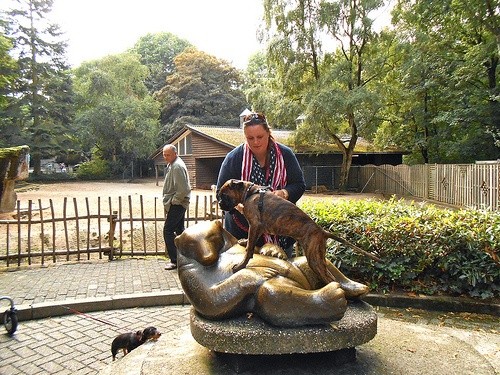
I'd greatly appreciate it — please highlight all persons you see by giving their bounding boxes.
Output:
[160,145,192,269]
[216,113,305,260]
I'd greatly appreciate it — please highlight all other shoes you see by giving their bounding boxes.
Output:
[165,264,177,270]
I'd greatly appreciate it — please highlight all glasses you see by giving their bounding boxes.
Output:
[244,114,265,122]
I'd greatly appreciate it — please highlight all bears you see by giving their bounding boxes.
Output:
[173,220,369,327]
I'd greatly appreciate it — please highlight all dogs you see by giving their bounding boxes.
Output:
[111,327,161,362]
[216,177,393,284]
